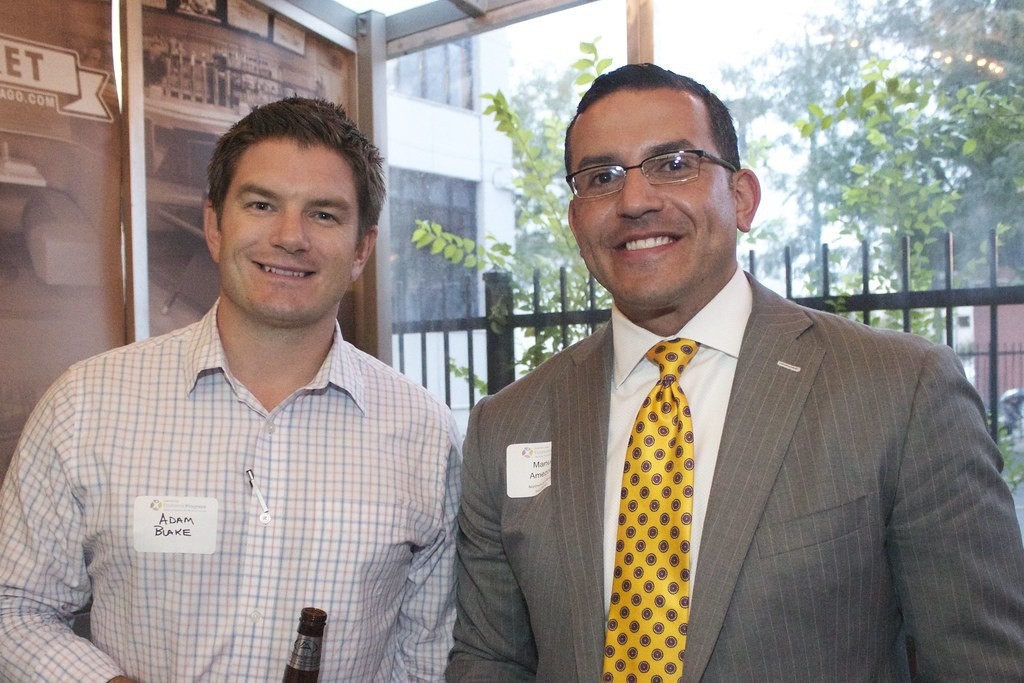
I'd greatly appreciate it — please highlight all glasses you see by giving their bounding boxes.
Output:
[566,150,739,198]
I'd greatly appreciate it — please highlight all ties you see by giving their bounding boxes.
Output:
[602,336,703,683]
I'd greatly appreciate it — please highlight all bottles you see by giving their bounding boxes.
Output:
[282,607,327,683]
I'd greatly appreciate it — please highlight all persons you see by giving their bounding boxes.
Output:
[444,64,1022,683]
[0,97,465,683]
